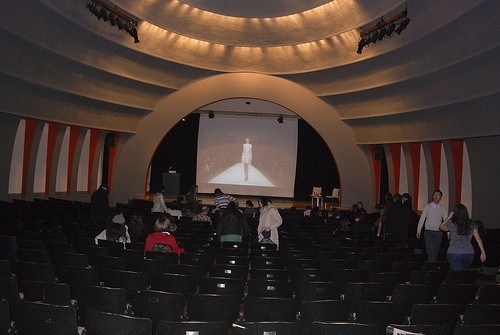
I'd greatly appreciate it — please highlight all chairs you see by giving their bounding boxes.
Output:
[323,188,340,207]
[310,187,325,208]
[0,196,499,335]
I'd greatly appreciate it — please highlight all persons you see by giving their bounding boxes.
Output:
[151,193,180,216]
[257,198,282,251]
[416,190,451,255]
[213,187,256,214]
[286,207,301,220]
[333,202,368,237]
[242,138,252,181]
[221,201,249,235]
[439,204,487,270]
[304,206,329,218]
[372,193,414,240]
[166,185,212,226]
[91,184,109,208]
[144,214,184,256]
[95,215,131,244]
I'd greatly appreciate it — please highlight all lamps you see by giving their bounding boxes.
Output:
[132,27,140,43]
[394,17,410,35]
[86,0,134,37]
[277,114,284,124]
[356,23,395,55]
[208,111,215,119]
